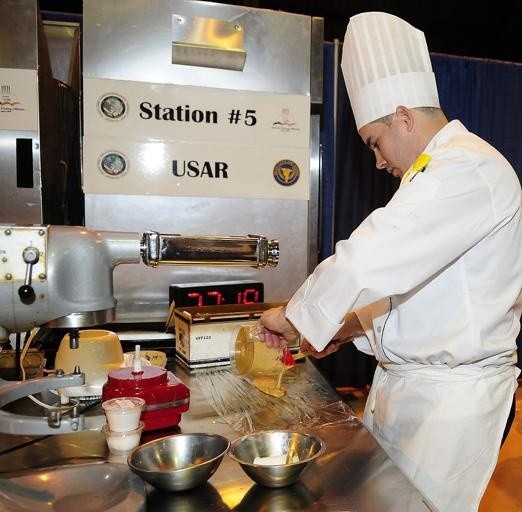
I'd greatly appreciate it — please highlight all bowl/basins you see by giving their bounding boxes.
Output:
[232,482,321,511]
[139,481,231,511]
[128,428,232,493]
[226,429,328,487]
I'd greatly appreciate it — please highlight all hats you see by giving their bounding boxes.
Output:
[340,11,441,133]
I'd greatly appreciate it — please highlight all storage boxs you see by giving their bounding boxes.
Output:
[174,301,306,370]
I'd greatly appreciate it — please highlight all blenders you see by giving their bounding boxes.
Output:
[0,220,280,507]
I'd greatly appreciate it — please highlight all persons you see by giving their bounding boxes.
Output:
[259,12,522,512]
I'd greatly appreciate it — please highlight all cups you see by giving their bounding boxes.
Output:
[100,394,145,433]
[227,323,288,377]
[102,423,146,456]
